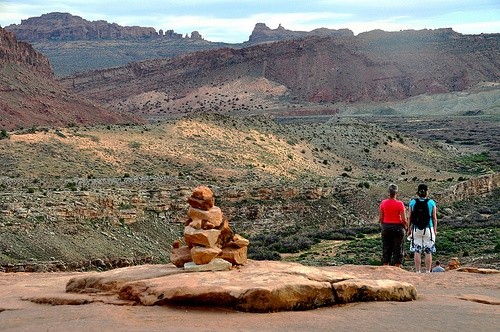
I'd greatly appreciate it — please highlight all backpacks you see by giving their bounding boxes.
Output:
[410,198,430,230]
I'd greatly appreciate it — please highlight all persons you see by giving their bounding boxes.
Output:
[433,261,445,272]
[407,184,438,274]
[379,184,407,268]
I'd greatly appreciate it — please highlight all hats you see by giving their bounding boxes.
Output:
[387,184,398,192]
[417,183,428,190]
